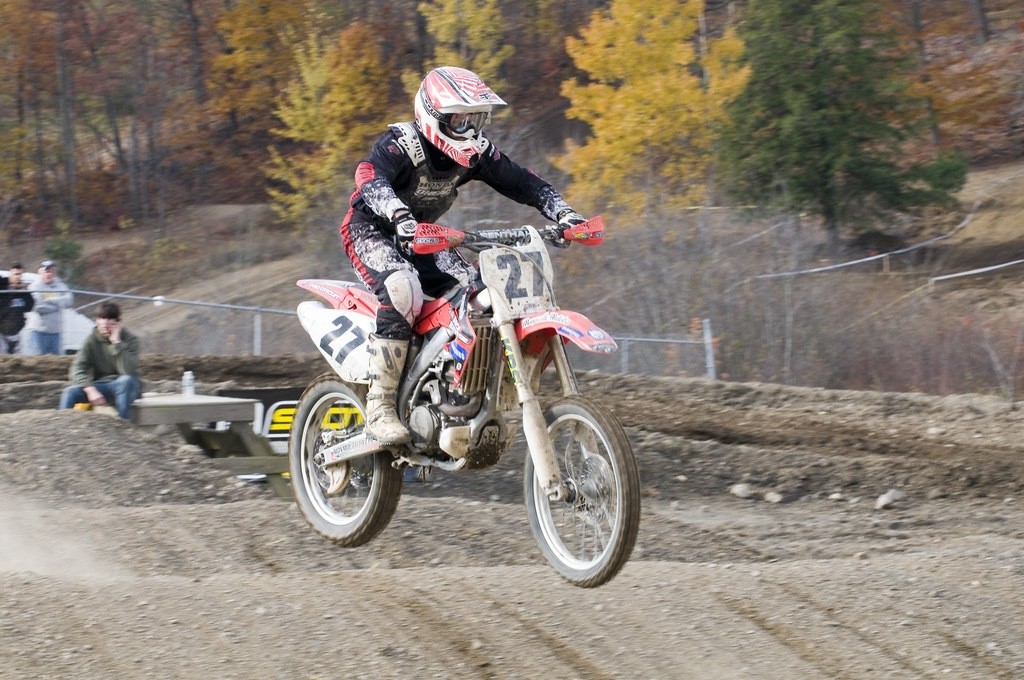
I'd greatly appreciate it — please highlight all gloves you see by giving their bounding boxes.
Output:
[392,212,424,256]
[554,207,596,242]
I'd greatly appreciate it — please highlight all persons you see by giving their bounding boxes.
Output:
[338,66,585,445]
[0,260,73,356]
[58,302,144,419]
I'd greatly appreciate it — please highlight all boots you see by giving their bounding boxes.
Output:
[364,332,413,446]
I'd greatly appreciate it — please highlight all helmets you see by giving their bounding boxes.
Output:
[415,66,508,168]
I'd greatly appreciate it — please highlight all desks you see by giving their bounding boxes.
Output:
[92,393,292,498]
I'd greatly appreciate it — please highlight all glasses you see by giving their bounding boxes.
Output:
[446,111,489,134]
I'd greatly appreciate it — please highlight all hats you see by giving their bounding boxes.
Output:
[39,261,57,272]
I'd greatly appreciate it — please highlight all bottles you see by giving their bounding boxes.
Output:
[182,372,195,403]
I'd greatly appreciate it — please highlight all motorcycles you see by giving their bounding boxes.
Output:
[287,216,641,590]
